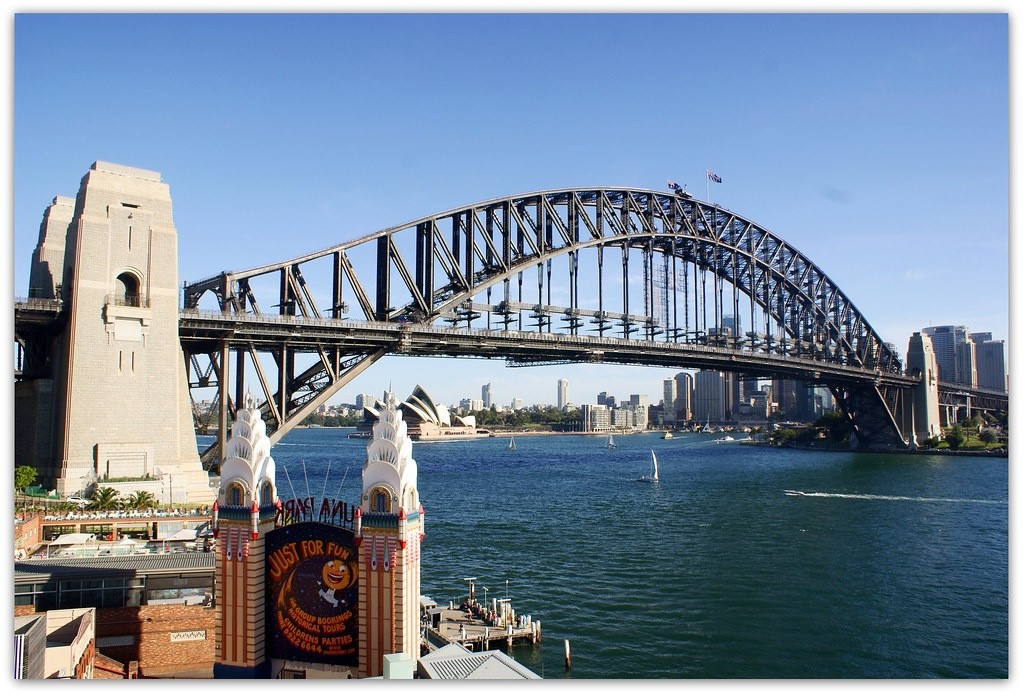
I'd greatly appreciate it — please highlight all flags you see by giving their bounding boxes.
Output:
[667,181,682,190]
[708,172,721,183]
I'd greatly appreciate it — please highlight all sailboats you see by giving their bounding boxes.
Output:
[506,435,517,450]
[605,433,617,449]
[638,449,659,483]
[662,430,674,440]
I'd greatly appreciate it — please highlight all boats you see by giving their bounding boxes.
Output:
[681,413,782,443]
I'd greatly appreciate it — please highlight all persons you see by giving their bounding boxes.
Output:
[458,599,527,635]
